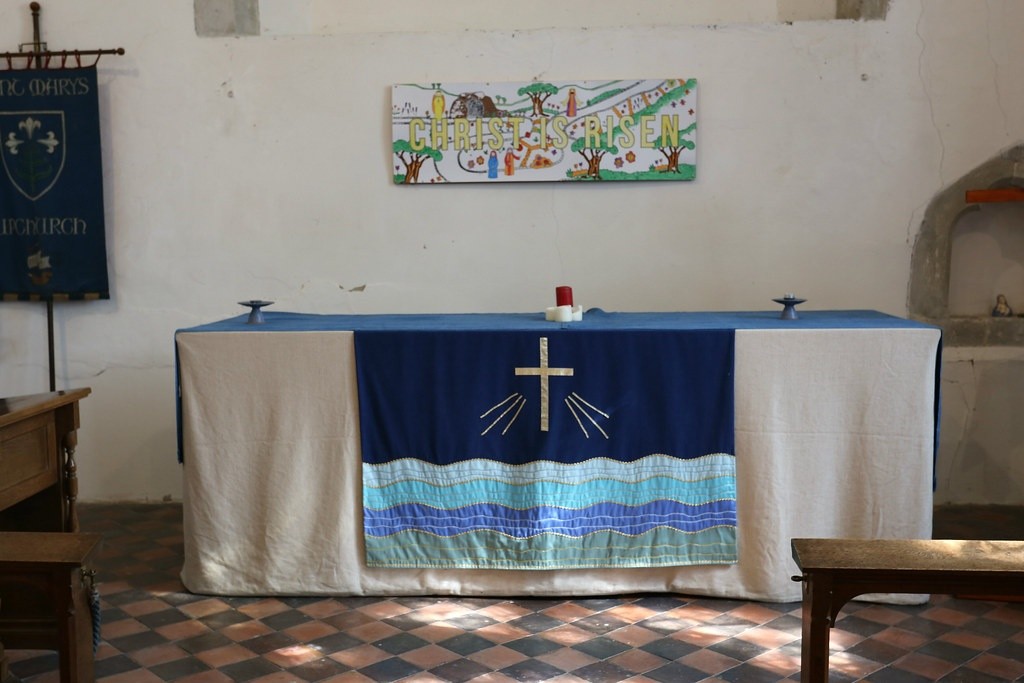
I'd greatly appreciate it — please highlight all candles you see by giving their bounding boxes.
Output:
[546,306,554,321]
[573,305,583,321]
[555,285,573,307]
[554,303,572,322]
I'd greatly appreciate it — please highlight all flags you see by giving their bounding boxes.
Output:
[0,65,110,303]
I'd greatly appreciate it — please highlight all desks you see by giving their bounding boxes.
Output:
[173,306,944,606]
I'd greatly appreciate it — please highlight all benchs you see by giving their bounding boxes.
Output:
[790,539,1023,683]
[0,531,102,683]
